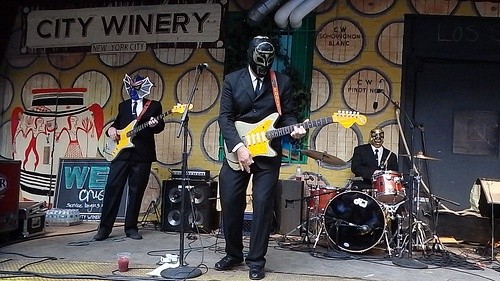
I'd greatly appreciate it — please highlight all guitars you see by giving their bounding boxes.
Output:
[103,103,195,162]
[224,110,368,171]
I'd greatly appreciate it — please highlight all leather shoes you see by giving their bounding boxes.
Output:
[249,264,265,281]
[214,254,244,272]
[93,226,112,241]
[124,228,142,240]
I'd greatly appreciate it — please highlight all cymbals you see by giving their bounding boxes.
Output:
[299,150,346,166]
[397,154,442,160]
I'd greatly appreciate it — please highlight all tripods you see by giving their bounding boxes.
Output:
[286,158,327,243]
[382,93,451,257]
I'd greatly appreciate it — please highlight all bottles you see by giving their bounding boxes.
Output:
[45,208,80,224]
[296,164,302,180]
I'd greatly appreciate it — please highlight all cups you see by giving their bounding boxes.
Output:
[117,252,132,272]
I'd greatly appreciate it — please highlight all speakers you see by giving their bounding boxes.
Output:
[161,179,218,234]
[474,178,500,218]
[276,179,304,236]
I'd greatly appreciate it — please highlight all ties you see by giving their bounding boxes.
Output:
[133,101,137,113]
[375,149,379,168]
[255,77,260,96]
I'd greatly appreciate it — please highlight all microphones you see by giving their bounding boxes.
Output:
[198,62,209,68]
[373,89,385,93]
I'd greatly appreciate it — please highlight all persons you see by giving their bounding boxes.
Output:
[93,75,165,241]
[215,36,309,280]
[351,128,402,237]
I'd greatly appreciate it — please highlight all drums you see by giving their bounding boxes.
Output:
[322,190,388,253]
[308,184,340,218]
[371,170,407,206]
